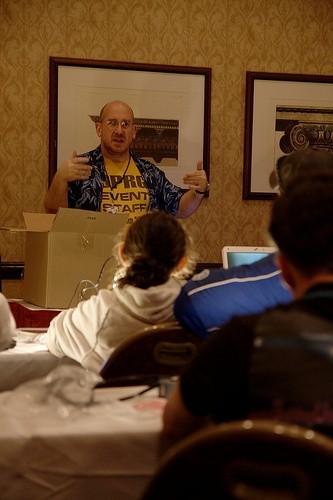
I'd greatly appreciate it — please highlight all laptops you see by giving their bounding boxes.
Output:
[221,245,278,274]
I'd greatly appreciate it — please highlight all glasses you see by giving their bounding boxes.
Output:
[100,119,132,129]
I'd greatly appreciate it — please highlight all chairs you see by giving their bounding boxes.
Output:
[93,321,200,389]
[140,407,333,500]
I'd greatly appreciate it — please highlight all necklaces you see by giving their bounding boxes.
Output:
[104,155,132,214]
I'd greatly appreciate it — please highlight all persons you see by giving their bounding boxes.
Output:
[46,100,207,220]
[160,176,333,450]
[47,211,196,373]
[282,148,333,189]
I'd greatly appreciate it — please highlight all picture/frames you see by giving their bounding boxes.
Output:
[46,55,212,199]
[241,70,333,200]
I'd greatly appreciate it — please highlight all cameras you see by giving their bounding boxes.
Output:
[158,376,179,397]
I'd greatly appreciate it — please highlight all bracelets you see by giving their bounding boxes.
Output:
[195,181,210,194]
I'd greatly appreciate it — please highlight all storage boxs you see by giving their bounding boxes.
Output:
[0,206,131,309]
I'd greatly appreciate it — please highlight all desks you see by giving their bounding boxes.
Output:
[0,327,169,500]
